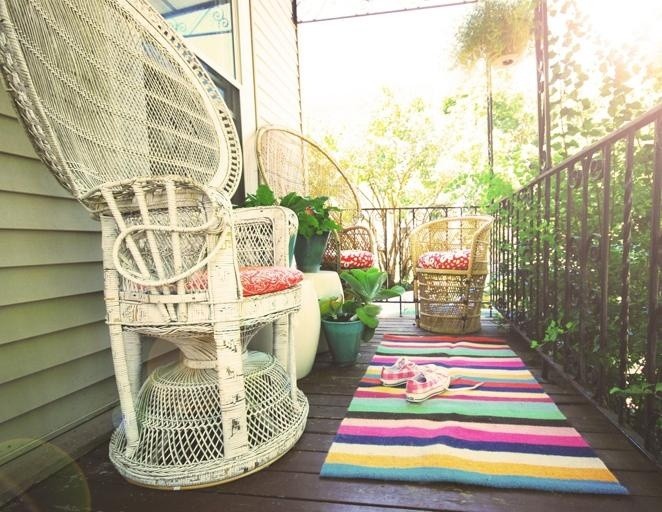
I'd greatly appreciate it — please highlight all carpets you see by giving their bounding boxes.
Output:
[318,329,629,498]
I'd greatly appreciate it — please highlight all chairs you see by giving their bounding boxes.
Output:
[253,122,380,281]
[0,0,310,492]
[402,212,495,336]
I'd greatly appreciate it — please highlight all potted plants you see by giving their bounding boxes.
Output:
[235,186,342,270]
[316,298,380,368]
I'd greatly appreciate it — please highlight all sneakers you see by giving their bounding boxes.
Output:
[379,355,436,388]
[405,366,451,403]
